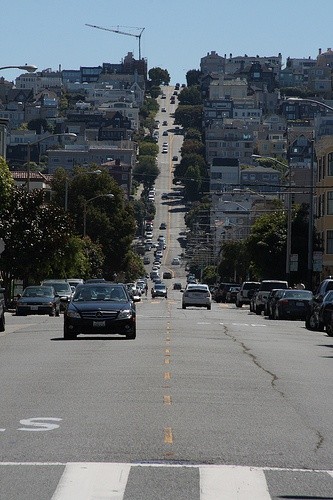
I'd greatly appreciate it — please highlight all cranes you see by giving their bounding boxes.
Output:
[85,23,145,60]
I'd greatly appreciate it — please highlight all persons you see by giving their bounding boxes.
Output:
[79,290,93,301]
[110,290,123,301]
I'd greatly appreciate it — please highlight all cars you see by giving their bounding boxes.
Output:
[162,81,186,104]
[153,120,168,153]
[16,279,146,316]
[161,107,166,111]
[163,258,196,289]
[59,283,136,340]
[180,284,212,310]
[144,222,167,300]
[210,279,333,336]
[173,156,178,160]
[0,279,6,331]
[149,187,156,203]
[174,126,180,134]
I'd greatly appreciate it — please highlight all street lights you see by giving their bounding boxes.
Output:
[64,169,101,235]
[84,194,114,256]
[224,200,249,281]
[202,220,238,282]
[26,132,77,193]
[251,154,292,284]
[233,188,267,215]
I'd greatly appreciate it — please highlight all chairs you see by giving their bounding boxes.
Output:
[111,290,120,298]
